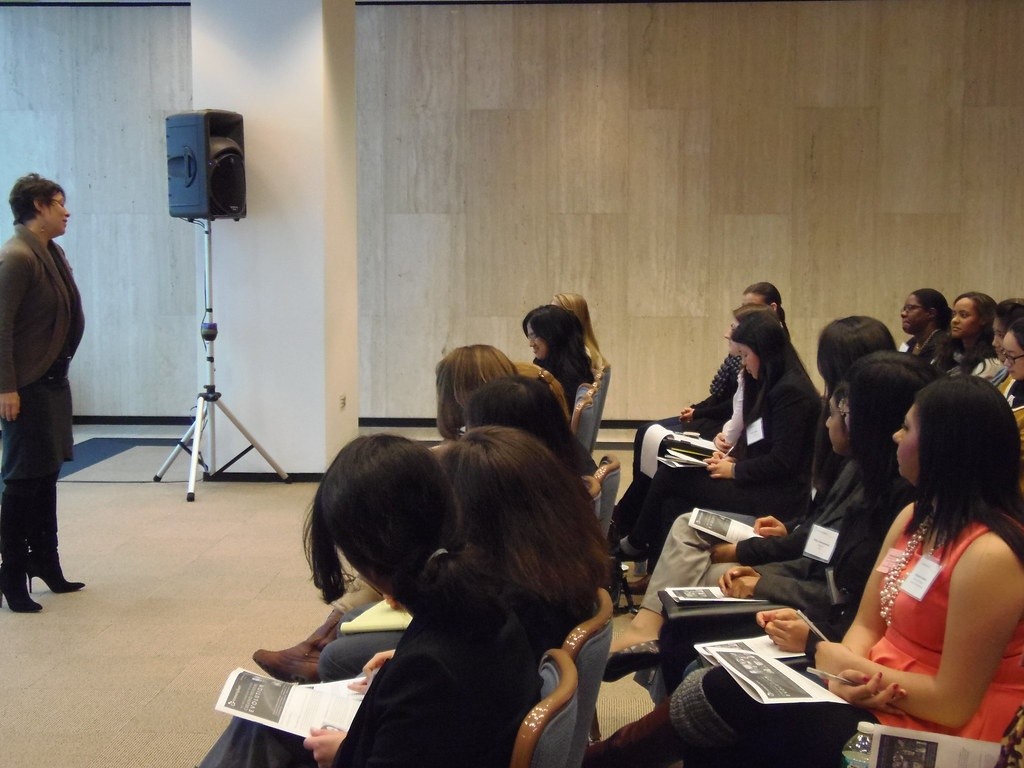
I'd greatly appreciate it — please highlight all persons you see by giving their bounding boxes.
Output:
[199,435,544,768]
[901,287,952,362]
[999,318,1024,495]
[0,173,87,611]
[252,294,604,684]
[611,281,945,692]
[319,425,612,680]
[581,376,1024,768]
[973,297,1024,387]
[936,292,997,372]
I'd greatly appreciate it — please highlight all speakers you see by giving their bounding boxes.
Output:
[164,109,247,222]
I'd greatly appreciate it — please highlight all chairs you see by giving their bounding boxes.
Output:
[571,360,639,615]
[510,586,613,768]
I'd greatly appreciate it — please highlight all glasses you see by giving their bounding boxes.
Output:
[838,399,852,415]
[828,405,838,417]
[900,303,927,313]
[526,333,541,341]
[1002,352,1023,365]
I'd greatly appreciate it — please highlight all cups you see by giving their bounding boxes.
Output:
[621,564,629,592]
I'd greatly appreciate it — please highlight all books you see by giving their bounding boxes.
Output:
[340,599,413,634]
[640,424,720,480]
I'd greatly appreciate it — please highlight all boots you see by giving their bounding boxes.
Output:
[252,607,350,684]
[582,666,723,768]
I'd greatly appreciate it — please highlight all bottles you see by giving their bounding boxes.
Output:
[841,721,874,768]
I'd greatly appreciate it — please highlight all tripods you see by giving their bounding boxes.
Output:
[153,219,292,501]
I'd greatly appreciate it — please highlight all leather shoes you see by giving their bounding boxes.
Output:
[613,536,649,563]
[623,572,654,596]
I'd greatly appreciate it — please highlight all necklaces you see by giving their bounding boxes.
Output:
[879,515,945,627]
[921,328,941,350]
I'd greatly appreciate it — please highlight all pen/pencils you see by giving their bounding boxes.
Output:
[806,667,859,687]
[796,609,831,642]
[721,447,734,459]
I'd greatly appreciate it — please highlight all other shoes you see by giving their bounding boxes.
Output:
[602,640,661,683]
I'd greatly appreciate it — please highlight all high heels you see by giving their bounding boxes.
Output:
[26,549,86,595]
[0,560,44,616]
